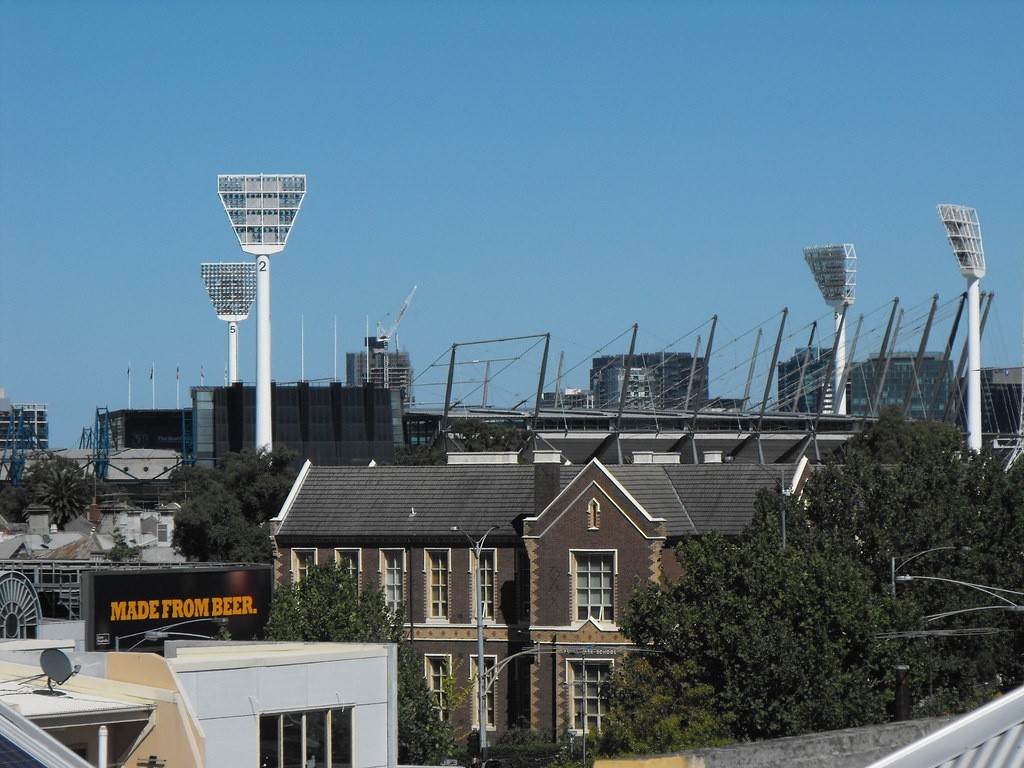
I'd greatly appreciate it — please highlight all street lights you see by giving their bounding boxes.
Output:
[891,546,973,604]
[448,524,503,753]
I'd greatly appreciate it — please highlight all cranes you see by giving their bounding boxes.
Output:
[375,284,417,388]
